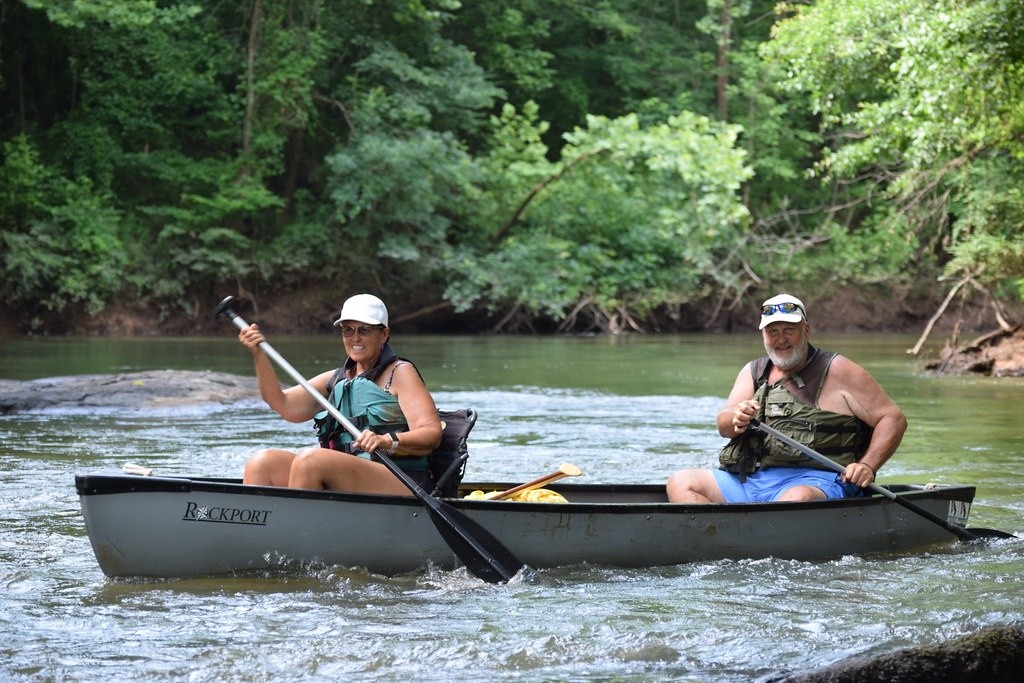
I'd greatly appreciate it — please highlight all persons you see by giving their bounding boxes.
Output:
[239,294,442,496]
[666,294,908,504]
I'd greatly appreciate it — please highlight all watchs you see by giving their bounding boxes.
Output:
[382,432,400,455]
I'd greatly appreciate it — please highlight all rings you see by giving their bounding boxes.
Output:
[248,337,253,341]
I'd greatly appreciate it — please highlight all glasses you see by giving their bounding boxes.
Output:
[761,302,807,321]
[341,325,385,338]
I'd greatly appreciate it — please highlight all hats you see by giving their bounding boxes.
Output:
[333,294,388,327]
[759,294,806,330]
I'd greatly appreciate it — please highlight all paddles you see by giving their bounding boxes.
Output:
[745,404,1019,545]
[207,291,525,589]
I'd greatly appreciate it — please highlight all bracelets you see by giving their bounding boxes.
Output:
[859,462,876,478]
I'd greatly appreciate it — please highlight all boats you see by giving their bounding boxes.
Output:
[76,472,977,580]
